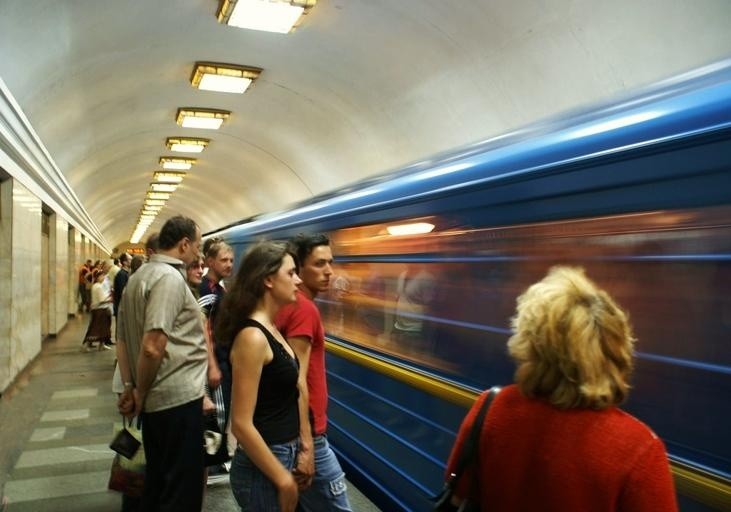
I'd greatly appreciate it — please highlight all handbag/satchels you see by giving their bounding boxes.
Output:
[106,422,146,498]
[430,475,478,511]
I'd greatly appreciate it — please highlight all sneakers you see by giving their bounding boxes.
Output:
[80,342,91,352]
[98,344,111,351]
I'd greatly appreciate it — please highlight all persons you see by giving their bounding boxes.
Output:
[446,265,680,511]
[324,262,436,361]
[79,217,352,511]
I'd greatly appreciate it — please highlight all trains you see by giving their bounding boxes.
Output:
[194,59,731,508]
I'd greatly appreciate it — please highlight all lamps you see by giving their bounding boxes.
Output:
[164,137,210,154]
[128,172,184,245]
[215,0,316,36]
[159,156,196,172]
[177,107,230,131]
[384,216,443,235]
[190,62,263,95]
